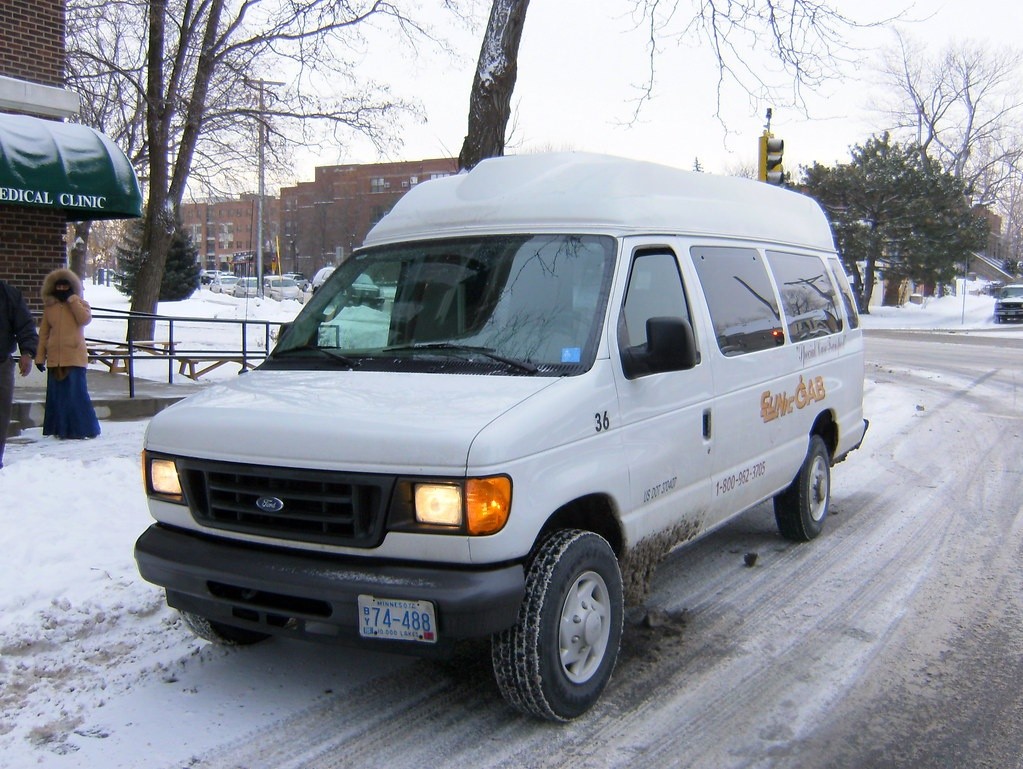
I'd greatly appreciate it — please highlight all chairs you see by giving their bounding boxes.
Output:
[412,280,468,342]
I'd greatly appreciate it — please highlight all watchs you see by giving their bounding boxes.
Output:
[23,352,33,357]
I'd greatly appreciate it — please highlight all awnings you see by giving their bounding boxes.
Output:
[0,113,142,219]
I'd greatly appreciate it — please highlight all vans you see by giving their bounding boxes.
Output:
[134,152,872,724]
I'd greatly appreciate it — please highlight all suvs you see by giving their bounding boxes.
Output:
[311,265,385,309]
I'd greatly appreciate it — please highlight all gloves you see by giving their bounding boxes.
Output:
[65,290,74,301]
[36,364,45,372]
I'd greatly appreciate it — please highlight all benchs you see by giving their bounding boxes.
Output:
[86,340,182,355]
[179,358,256,381]
[87,347,136,374]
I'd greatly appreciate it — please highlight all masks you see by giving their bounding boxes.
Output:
[54,290,71,298]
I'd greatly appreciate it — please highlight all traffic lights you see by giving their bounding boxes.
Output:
[757,130,786,189]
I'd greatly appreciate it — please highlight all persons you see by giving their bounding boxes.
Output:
[35,269,101,438]
[0,282,39,468]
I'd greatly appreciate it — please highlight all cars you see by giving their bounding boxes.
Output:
[255,276,305,305]
[199,269,234,285]
[208,276,238,296]
[232,277,257,299]
[281,272,309,293]
[993,283,1023,324]
